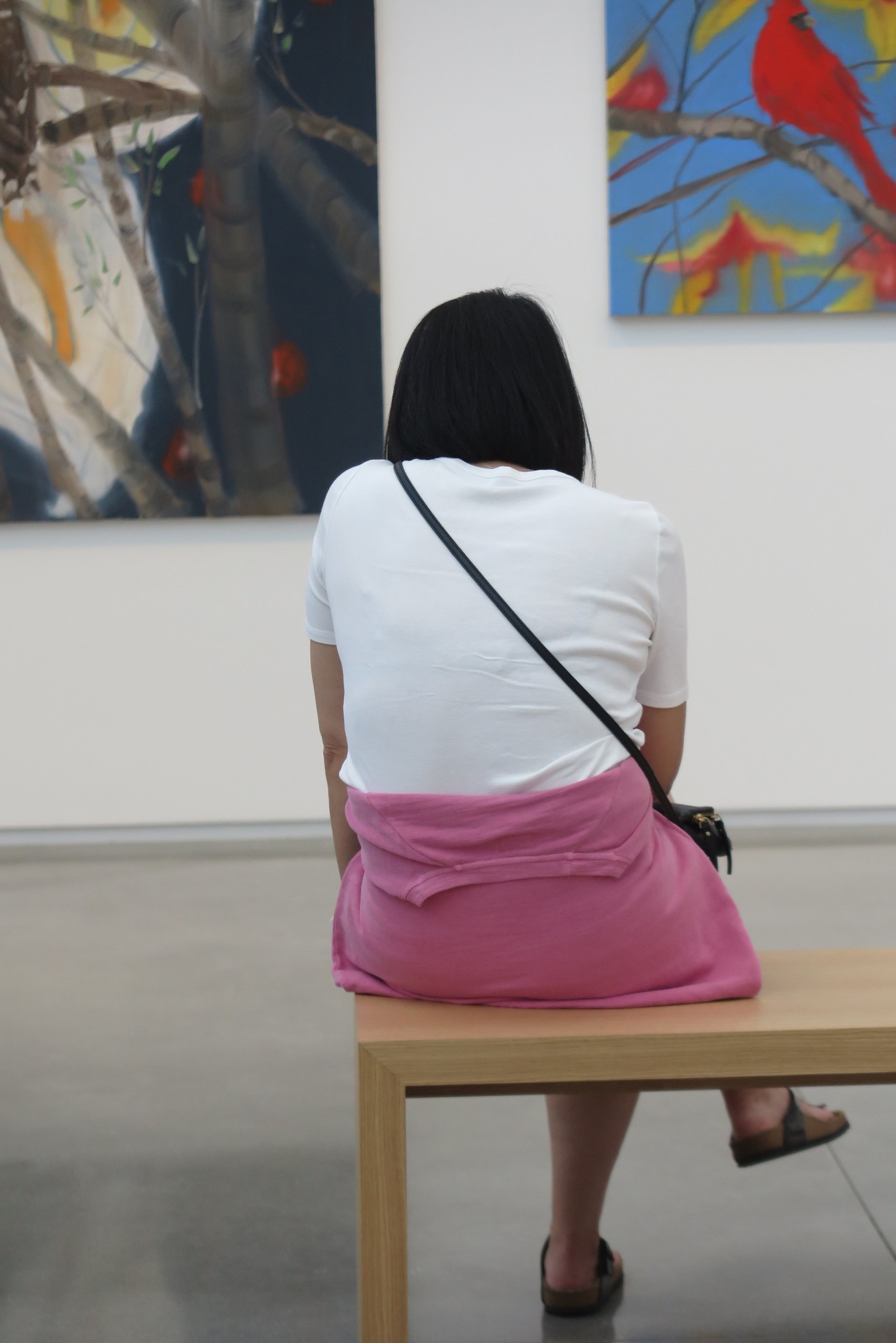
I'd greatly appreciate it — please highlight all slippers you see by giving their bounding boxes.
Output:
[537,1232,622,1315]
[730,1085,849,1169]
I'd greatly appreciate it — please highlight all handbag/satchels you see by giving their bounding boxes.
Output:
[656,798,734,880]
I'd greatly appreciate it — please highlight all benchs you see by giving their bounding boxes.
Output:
[356,947,896,1343]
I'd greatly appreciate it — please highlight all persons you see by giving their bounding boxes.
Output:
[302,288,849,1314]
